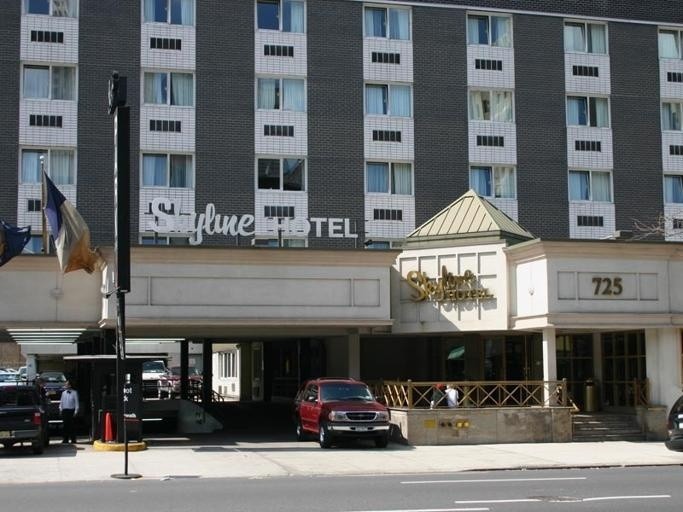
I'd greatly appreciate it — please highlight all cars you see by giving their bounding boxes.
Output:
[0,366,68,454]
[665,396,683,452]
[156,366,203,399]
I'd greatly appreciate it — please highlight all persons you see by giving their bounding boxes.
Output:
[429,385,448,410]
[59,379,80,444]
[445,386,460,408]
[34,379,50,446]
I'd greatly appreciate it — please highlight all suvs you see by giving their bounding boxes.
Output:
[288,377,390,448]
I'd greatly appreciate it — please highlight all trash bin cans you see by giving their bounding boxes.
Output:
[584,380,596,412]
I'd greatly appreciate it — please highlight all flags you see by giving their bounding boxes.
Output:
[41,166,102,277]
[0,221,32,268]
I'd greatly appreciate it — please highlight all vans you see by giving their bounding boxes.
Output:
[126,360,167,398]
